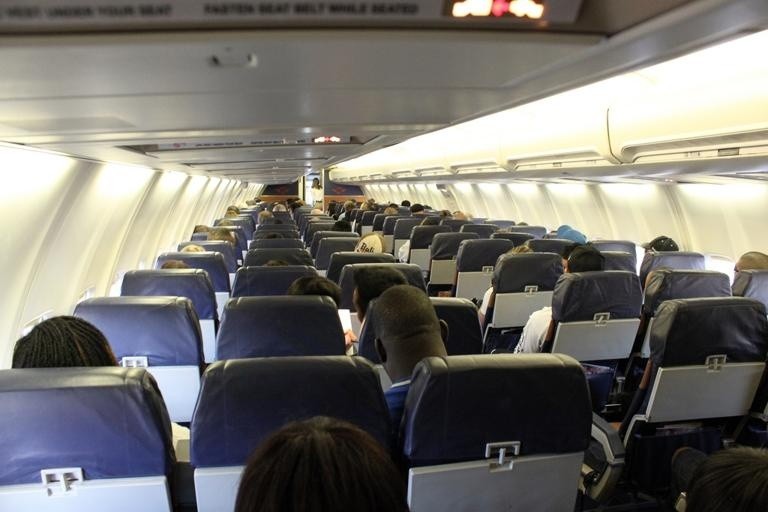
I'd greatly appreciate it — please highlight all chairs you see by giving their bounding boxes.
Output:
[383,216,416,253]
[315,237,361,276]
[191,232,244,267]
[243,248,312,266]
[578,297,768,511]
[231,265,318,296]
[543,272,641,415]
[156,252,231,322]
[426,233,479,298]
[484,221,515,228]
[0,366,177,512]
[339,263,427,309]
[642,270,732,398]
[527,239,572,258]
[178,241,238,289]
[310,231,360,256]
[511,226,546,236]
[640,252,704,280]
[122,269,216,364]
[394,219,419,263]
[210,226,248,251]
[455,239,513,299]
[491,234,534,246]
[190,356,395,512]
[206,201,352,239]
[358,297,484,357]
[590,241,635,251]
[327,252,394,279]
[731,269,768,315]
[249,239,302,249]
[408,226,452,277]
[399,353,593,512]
[215,295,346,361]
[362,211,377,236]
[461,224,497,239]
[440,220,473,232]
[483,253,563,354]
[73,295,207,424]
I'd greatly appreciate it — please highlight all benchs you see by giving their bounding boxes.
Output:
[599,251,634,271]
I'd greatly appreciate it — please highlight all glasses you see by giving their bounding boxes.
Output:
[733,265,739,273]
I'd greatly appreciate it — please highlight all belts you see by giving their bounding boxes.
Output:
[315,201,322,204]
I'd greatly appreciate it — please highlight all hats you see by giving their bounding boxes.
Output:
[313,179,318,182]
[410,204,424,212]
[557,225,586,245]
[641,236,679,252]
[354,233,386,253]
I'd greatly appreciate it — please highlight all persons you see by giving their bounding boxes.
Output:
[733,253,768,269]
[283,273,359,357]
[231,416,407,512]
[367,284,452,391]
[510,245,612,354]
[636,233,679,287]
[561,242,593,275]
[11,318,118,370]
[687,448,768,512]
[156,177,564,269]
[350,263,410,327]
